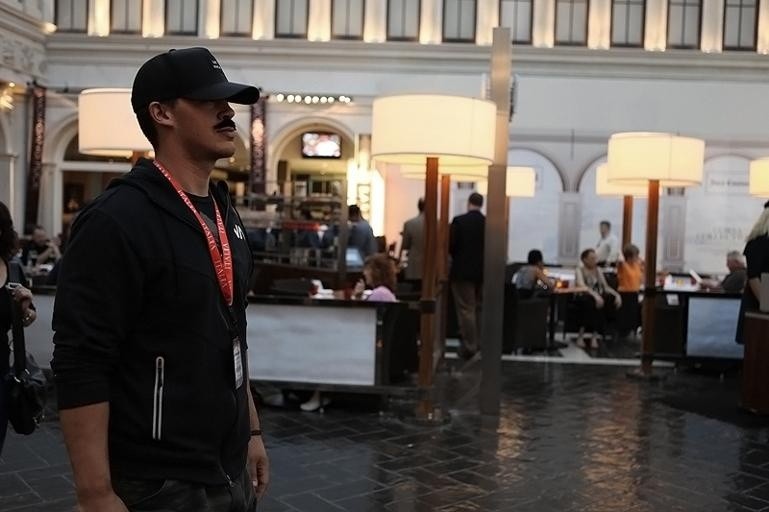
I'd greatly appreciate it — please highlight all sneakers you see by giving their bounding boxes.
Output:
[301,397,332,411]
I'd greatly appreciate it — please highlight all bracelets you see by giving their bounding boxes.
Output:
[250,430,262,436]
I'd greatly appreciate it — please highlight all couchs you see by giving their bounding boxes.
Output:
[569,272,617,345]
[502,281,548,356]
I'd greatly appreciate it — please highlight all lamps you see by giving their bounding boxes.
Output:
[77,86,155,168]
[365,96,496,427]
[594,165,664,252]
[481,166,537,269]
[611,129,707,378]
[749,156,767,199]
[403,164,487,366]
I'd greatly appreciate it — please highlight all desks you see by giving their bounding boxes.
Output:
[534,283,579,343]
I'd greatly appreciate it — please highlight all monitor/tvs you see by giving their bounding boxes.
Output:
[299,131,342,158]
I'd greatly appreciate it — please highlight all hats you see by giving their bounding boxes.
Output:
[131,46,259,113]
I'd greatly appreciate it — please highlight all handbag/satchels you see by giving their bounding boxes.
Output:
[5,351,50,435]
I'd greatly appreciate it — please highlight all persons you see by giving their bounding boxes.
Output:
[20,225,56,266]
[689,250,749,293]
[270,202,379,255]
[449,192,489,361]
[574,248,622,350]
[1,200,32,462]
[245,197,269,254]
[736,199,768,415]
[617,244,671,293]
[50,48,270,512]
[594,220,620,263]
[299,254,399,414]
[513,250,557,355]
[399,199,428,282]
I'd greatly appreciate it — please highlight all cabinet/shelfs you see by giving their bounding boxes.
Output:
[254,173,351,280]
